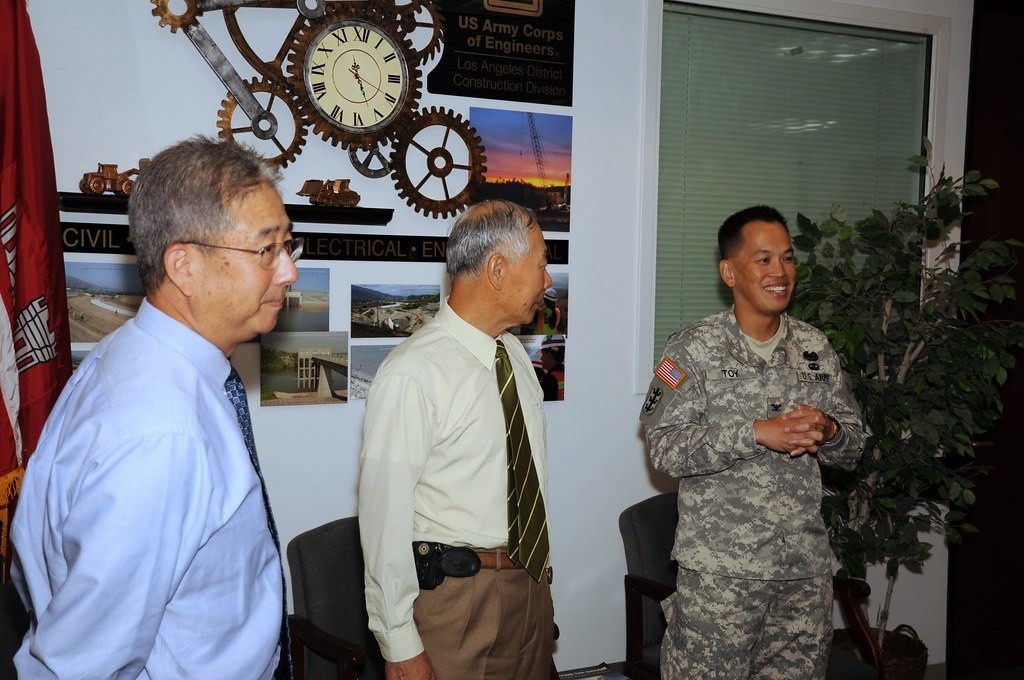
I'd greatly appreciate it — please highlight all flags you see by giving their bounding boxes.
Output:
[0,0,74,580]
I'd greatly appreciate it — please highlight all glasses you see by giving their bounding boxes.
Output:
[173,238,304,271]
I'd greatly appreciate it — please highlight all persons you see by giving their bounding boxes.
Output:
[641,204,867,680]
[9,140,295,680]
[359,201,554,680]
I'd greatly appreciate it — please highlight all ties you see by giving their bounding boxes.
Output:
[225,367,293,680]
[495,340,550,583]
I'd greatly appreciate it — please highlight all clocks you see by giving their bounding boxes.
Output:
[286,10,421,149]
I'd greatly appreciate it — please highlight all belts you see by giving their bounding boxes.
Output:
[472,552,524,569]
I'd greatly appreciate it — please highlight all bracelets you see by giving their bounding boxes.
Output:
[826,413,840,437]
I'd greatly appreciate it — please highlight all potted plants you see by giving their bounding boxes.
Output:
[787,138,1024,680]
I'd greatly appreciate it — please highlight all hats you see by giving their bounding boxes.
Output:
[531,360,543,368]
[543,288,557,301]
[536,336,565,354]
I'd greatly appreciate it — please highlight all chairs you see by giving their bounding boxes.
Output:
[286,518,560,680]
[619,491,885,680]
